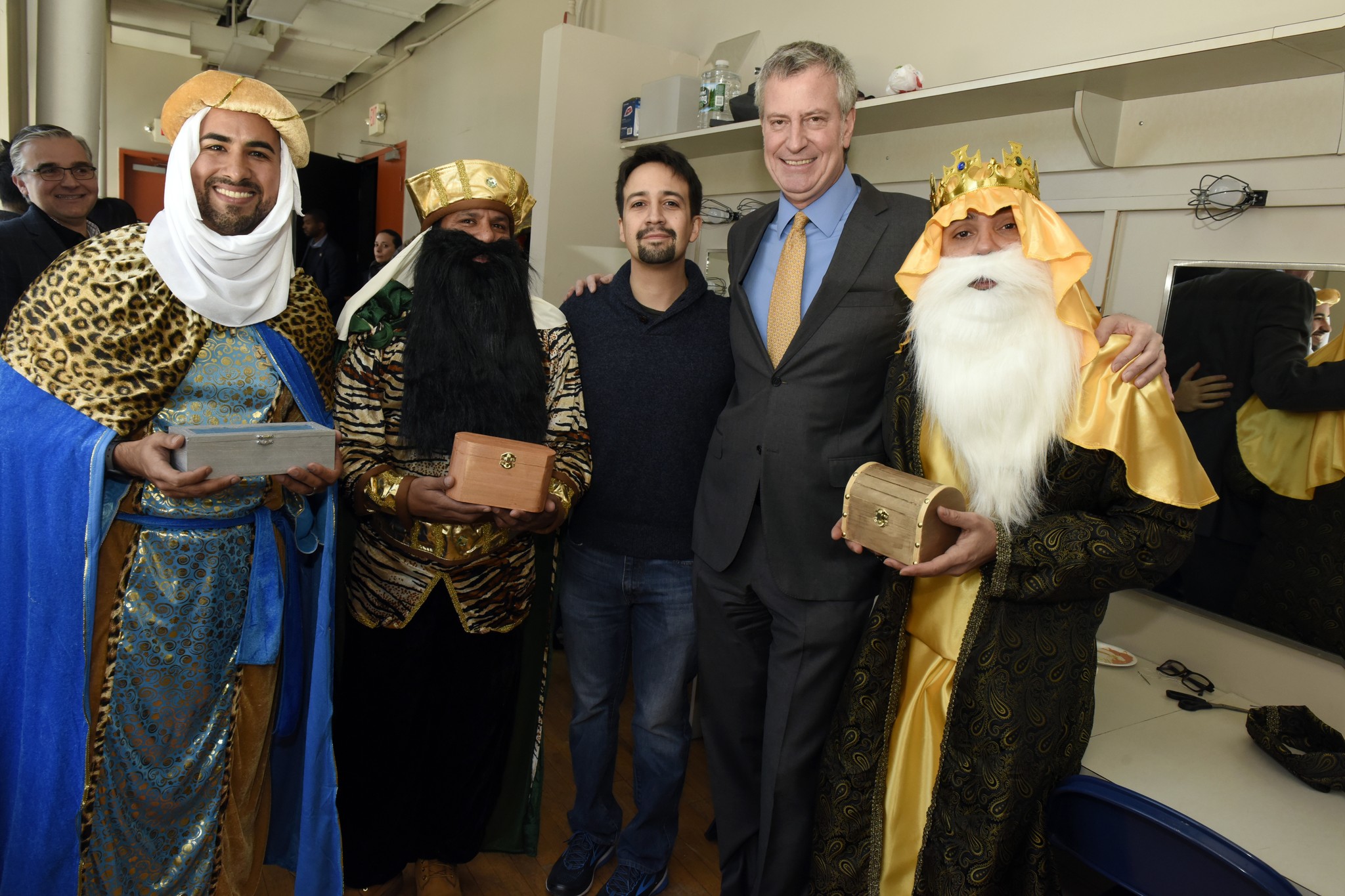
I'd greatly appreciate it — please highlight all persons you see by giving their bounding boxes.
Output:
[1130,266,1344,656]
[564,42,1178,896]
[1,70,343,896]
[0,124,1220,896]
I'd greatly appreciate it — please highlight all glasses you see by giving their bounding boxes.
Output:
[21,165,96,181]
[1157,660,1214,696]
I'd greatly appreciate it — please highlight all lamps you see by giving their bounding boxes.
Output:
[364,103,387,136]
[1188,174,1268,221]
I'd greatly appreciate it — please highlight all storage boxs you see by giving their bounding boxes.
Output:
[638,31,762,138]
[620,97,641,140]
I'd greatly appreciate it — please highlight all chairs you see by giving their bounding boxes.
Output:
[1042,774,1303,896]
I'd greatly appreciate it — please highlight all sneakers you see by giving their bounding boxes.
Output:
[596,864,669,896]
[415,857,462,896]
[546,831,614,896]
[344,874,402,895]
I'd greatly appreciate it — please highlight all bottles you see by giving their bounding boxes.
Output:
[696,60,742,129]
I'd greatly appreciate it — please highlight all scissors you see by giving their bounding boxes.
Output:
[1166,689,1248,714]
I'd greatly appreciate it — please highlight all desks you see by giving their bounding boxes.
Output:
[1084,650,1345,896]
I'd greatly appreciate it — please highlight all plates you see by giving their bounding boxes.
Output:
[1097,641,1138,667]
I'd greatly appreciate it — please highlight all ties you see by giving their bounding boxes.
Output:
[767,211,811,370]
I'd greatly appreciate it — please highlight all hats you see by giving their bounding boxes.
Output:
[401,158,537,240]
[160,70,310,168]
